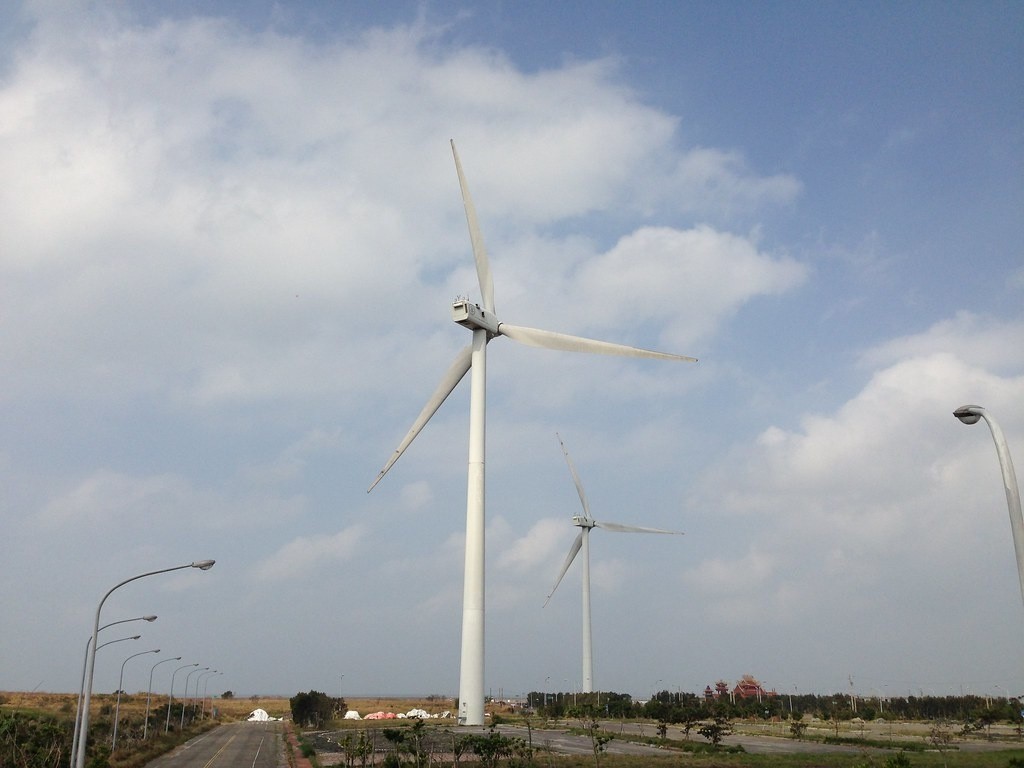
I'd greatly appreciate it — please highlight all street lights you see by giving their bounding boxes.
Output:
[181,667,210,728]
[191,670,218,724]
[143,655,182,739]
[339,674,344,713]
[201,672,224,716]
[70,613,157,768]
[112,649,160,752]
[164,663,200,735]
[73,558,216,768]
[953,403,1023,608]
